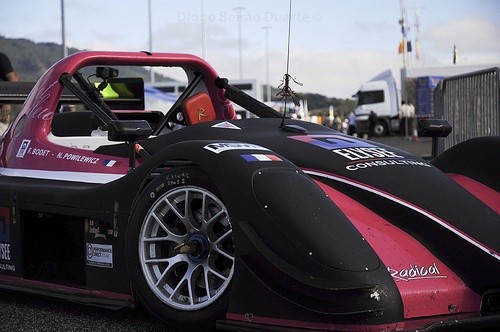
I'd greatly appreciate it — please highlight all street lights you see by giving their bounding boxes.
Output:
[232,5,246,80]
[259,25,275,103]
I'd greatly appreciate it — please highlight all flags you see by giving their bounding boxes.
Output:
[398,19,411,53]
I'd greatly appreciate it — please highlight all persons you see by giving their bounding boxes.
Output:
[0,52,18,138]
[401,100,420,142]
[315,111,357,136]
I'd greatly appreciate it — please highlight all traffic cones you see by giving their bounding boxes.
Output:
[411,125,419,142]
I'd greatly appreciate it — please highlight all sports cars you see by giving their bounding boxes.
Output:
[0,50,499,332]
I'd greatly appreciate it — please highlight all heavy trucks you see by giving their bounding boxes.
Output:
[352,68,441,135]
